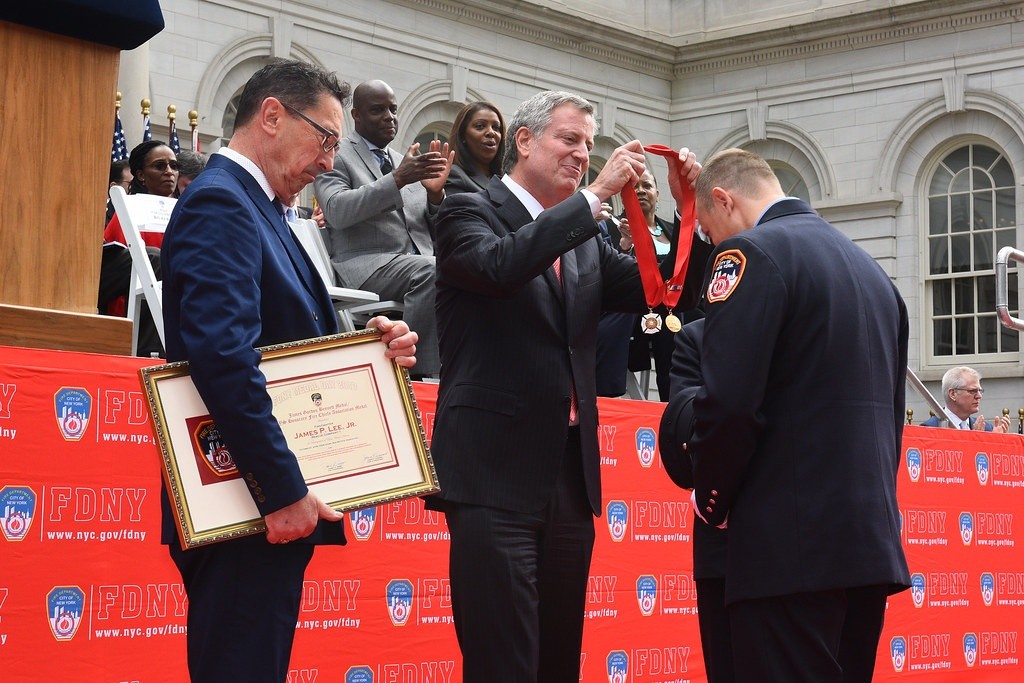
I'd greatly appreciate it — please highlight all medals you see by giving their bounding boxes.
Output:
[666,314,681,333]
[641,312,662,334]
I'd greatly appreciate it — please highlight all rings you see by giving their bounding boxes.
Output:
[279,538,291,543]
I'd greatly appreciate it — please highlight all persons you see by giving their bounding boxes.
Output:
[919,366,1010,434]
[98,79,712,402]
[689,148,914,683]
[162,57,418,683]
[419,88,702,683]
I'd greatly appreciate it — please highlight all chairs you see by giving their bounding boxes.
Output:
[108,186,647,402]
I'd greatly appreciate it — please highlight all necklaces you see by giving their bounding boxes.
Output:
[646,222,663,236]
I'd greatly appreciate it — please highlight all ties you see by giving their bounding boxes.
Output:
[372,150,392,175]
[553,256,578,422]
[272,196,289,232]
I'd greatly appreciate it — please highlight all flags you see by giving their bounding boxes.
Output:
[112,112,131,162]
[143,113,155,143]
[169,118,180,156]
[192,128,201,153]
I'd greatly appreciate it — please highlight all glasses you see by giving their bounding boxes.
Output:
[141,161,180,171]
[281,102,340,153]
[956,388,984,396]
